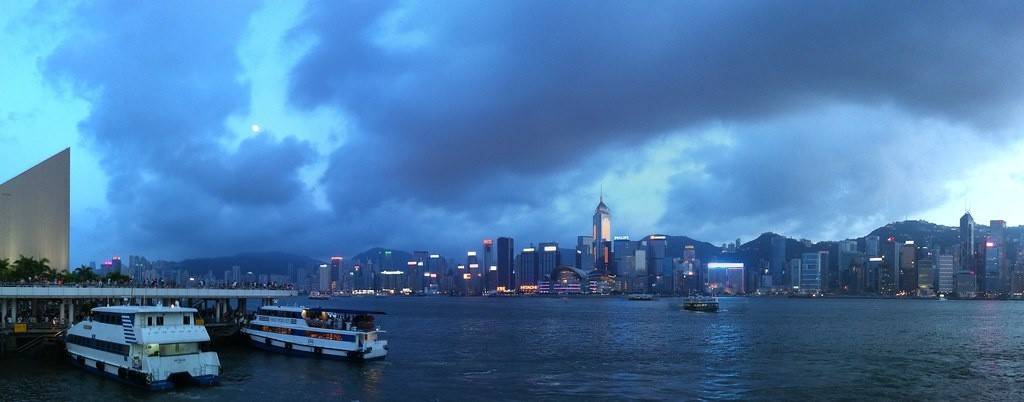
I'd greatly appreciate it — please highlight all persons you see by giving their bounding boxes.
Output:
[20,275,293,291]
[4,298,248,328]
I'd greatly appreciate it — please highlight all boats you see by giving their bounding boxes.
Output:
[66,301,223,394]
[681,286,720,311]
[331,290,352,297]
[239,303,390,364]
[375,292,389,298]
[629,293,653,301]
[309,290,330,300]
